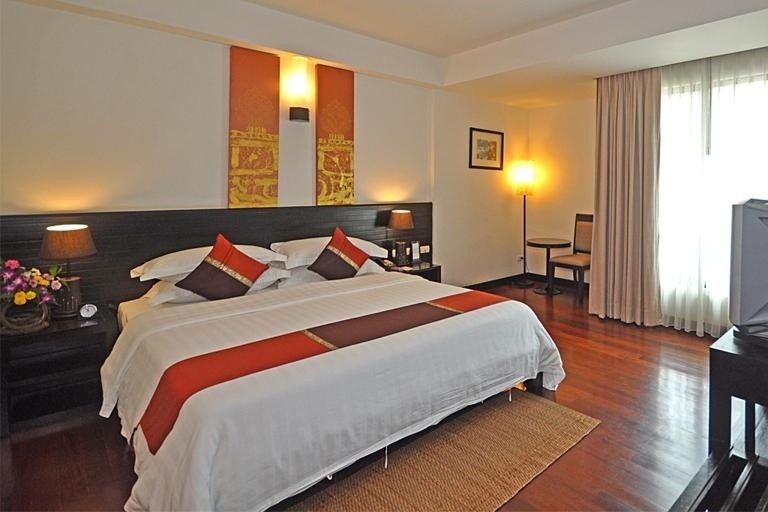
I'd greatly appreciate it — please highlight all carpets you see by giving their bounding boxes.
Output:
[281,389,601,512]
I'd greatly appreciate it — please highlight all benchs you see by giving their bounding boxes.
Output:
[667,440,768,512]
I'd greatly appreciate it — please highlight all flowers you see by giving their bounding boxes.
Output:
[0,258,71,307]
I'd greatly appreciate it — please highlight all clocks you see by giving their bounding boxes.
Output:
[79,303,98,321]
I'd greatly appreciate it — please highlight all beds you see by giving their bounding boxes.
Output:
[96,271,566,511]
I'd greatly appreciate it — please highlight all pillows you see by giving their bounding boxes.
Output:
[129,225,388,307]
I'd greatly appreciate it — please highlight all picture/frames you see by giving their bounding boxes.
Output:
[468,125,504,171]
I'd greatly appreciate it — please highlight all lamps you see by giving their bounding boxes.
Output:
[511,159,538,289]
[39,224,98,319]
[387,209,414,266]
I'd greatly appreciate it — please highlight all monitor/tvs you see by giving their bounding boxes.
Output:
[728,197,768,340]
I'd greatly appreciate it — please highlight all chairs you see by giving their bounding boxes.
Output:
[548,213,593,303]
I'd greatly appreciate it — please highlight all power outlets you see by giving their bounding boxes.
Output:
[517,255,524,263]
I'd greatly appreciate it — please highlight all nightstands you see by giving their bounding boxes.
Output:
[0,307,118,438]
[397,259,441,283]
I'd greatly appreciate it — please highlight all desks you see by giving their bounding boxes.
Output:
[709,326,768,462]
[527,237,571,296]
